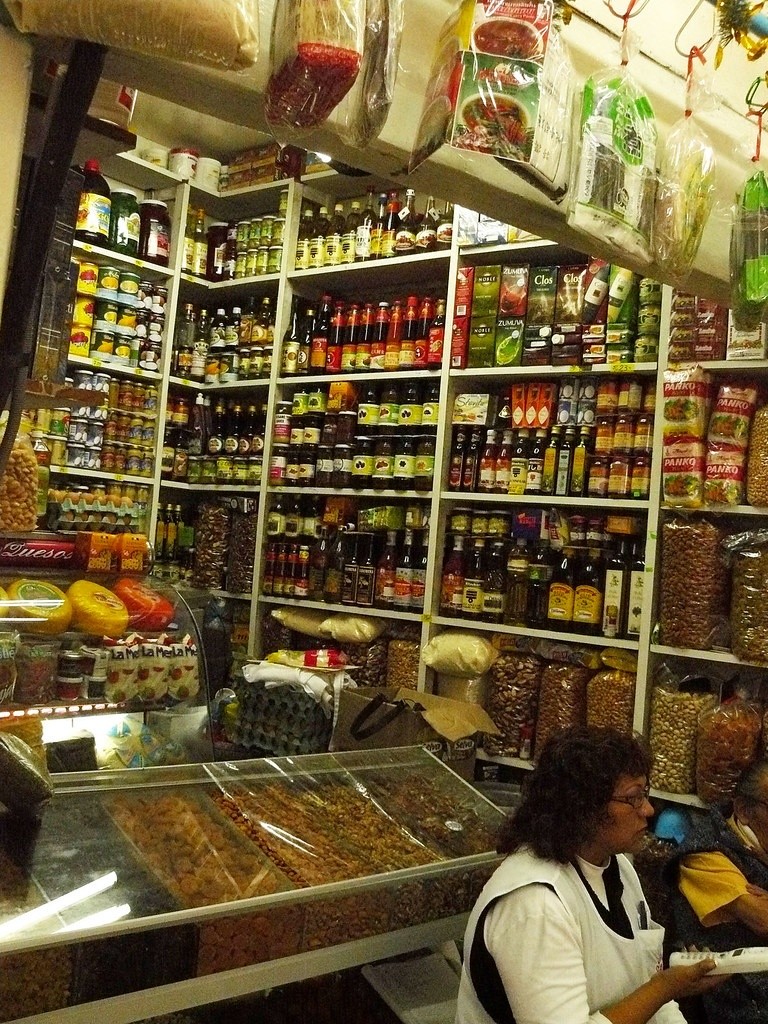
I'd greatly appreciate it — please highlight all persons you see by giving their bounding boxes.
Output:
[658,757,768,1024]
[454,724,733,1024]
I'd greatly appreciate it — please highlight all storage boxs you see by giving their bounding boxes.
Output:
[324,497,359,524]
[326,382,368,415]
[71,532,116,573]
[334,687,502,783]
[449,256,609,369]
[357,507,405,529]
[114,533,146,572]
[229,145,278,191]
[511,382,559,429]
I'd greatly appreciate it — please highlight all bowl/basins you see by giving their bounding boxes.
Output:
[472,18,542,60]
[461,92,530,135]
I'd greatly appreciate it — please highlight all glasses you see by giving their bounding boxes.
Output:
[610,785,650,809]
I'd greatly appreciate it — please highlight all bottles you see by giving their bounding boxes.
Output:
[309,525,429,614]
[153,503,186,561]
[280,294,446,375]
[450,426,587,495]
[189,393,266,456]
[294,186,452,270]
[439,535,649,639]
[181,208,208,278]
[175,295,276,345]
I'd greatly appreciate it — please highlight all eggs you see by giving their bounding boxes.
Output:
[46,489,134,525]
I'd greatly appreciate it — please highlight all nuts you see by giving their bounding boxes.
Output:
[205,780,461,952]
[480,404,767,795]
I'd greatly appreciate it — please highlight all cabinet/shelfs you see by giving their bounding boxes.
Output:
[47,154,220,575]
[190,167,767,810]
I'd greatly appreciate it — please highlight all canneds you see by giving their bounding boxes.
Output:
[182,212,358,490]
[19,188,174,477]
